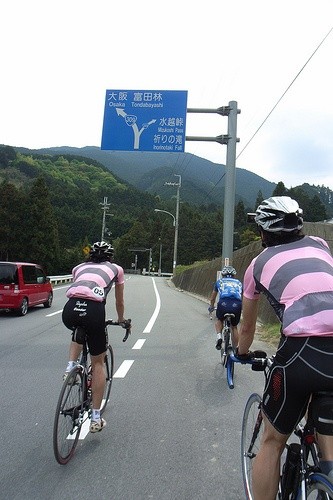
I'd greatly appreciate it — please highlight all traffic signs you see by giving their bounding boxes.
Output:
[101,89,189,153]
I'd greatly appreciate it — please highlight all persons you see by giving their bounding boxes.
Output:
[237,197,333,500]
[62,242,126,432]
[210,267,243,350]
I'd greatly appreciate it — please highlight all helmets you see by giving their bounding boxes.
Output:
[221,266,237,275]
[90,241,114,257]
[254,196,303,232]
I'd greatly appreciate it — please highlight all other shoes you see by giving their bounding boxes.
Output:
[62,373,79,384]
[215,338,222,350]
[89,418,106,433]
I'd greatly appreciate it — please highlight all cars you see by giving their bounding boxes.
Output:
[0,261,54,316]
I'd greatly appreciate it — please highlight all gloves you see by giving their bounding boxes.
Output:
[234,346,255,364]
[208,306,215,312]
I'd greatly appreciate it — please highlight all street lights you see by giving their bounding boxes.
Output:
[154,209,178,273]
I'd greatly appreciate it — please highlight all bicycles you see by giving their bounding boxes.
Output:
[209,307,236,368]
[53,318,132,465]
[227,350,333,500]
